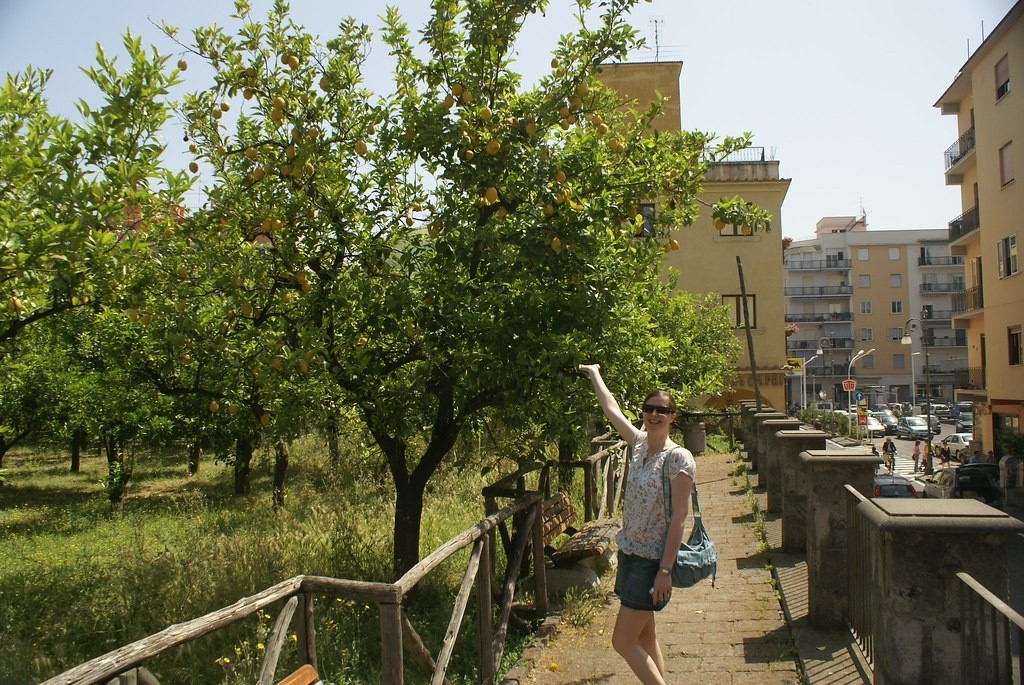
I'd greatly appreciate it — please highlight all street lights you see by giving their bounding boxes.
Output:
[847,347,877,437]
[900,310,934,476]
[800,355,818,418]
[815,332,839,437]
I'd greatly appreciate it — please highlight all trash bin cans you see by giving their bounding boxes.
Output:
[682,422,707,453]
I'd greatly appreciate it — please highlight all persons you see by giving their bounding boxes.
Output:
[913,441,921,472]
[924,440,935,467]
[970,450,996,464]
[892,405,900,419]
[579,363,695,685]
[872,447,880,477]
[940,442,951,470]
[883,437,897,467]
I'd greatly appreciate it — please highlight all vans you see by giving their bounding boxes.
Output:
[922,463,1005,510]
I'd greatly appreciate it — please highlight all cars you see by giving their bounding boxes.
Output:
[873,475,919,499]
[833,398,973,440]
[935,433,975,460]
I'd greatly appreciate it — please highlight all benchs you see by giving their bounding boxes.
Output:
[523,489,624,566]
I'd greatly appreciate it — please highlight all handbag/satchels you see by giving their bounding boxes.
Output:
[663,516,717,588]
[912,454,915,460]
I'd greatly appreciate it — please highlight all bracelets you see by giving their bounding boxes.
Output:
[659,568,673,575]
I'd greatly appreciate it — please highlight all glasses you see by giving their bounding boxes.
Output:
[642,404,673,414]
